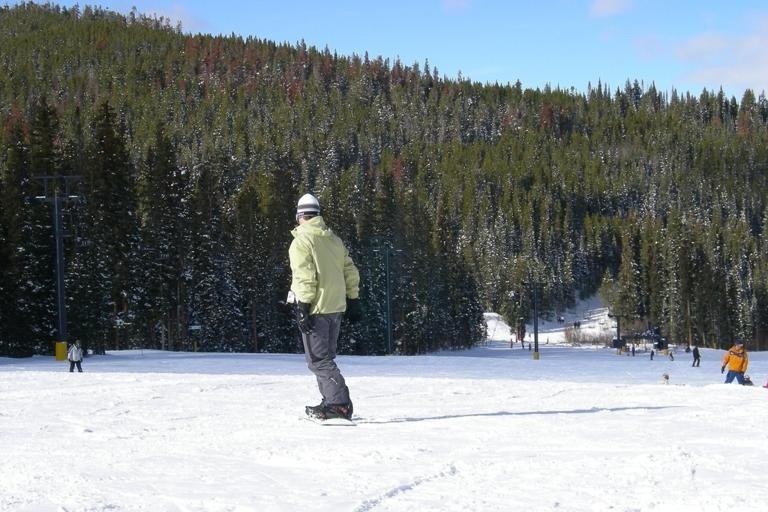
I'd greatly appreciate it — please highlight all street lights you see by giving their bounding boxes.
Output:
[369,237,403,354]
[32,172,82,359]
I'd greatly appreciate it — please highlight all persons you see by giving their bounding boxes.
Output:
[720,339,749,385]
[686,345,690,352]
[67,339,84,372]
[573,320,577,329]
[691,346,701,367]
[278,193,362,420]
[650,350,655,360]
[670,351,674,361]
[576,320,581,329]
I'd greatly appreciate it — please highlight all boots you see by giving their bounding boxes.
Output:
[309,400,354,420]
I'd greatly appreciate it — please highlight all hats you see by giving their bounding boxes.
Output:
[295,193,321,213]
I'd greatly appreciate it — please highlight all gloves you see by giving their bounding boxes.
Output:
[295,301,314,335]
[343,296,363,324]
